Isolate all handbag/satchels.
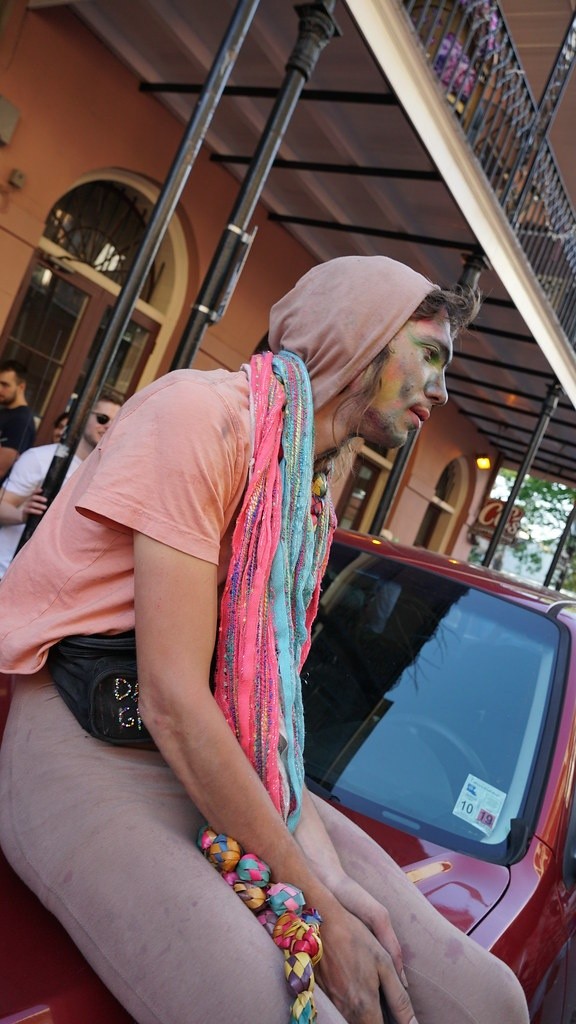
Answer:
[43,630,221,749]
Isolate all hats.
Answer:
[267,254,442,415]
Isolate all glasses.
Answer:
[91,410,113,424]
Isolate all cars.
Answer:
[0,528,576,1023]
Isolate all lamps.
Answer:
[475,453,491,469]
[35,267,54,289]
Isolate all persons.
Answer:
[0,356,127,581]
[0,255,533,1024]
